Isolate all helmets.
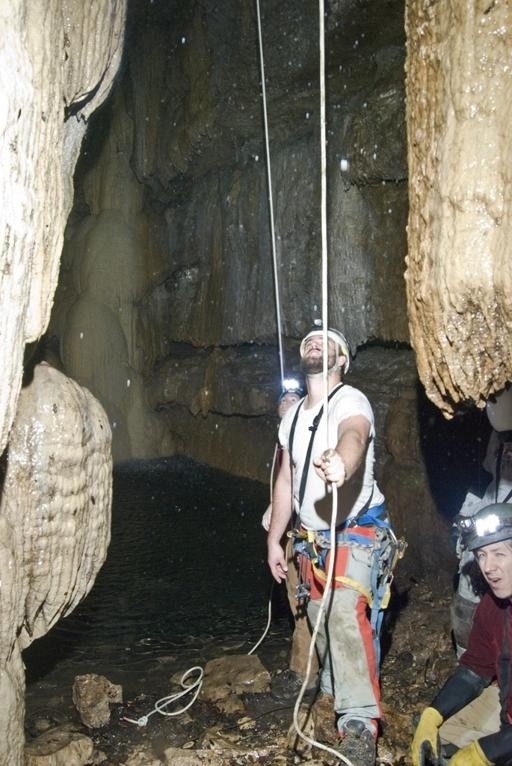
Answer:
[280,387,305,399]
[299,327,351,375]
[451,503,512,551]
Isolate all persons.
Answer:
[266,327,409,766]
[411,502,512,766]
[451,426,511,665]
[261,378,320,702]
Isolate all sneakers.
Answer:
[339,712,378,765]
[313,693,338,743]
[287,703,315,753]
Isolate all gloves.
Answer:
[448,739,496,766]
[410,707,443,766]
